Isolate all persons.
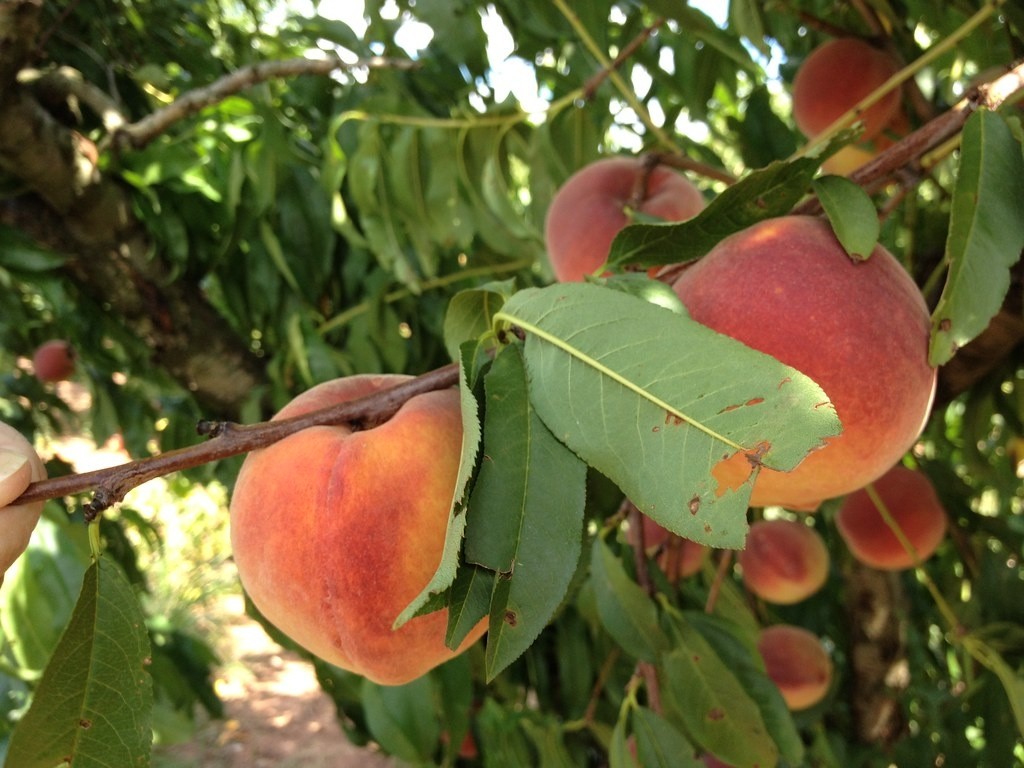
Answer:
[0,420,48,592]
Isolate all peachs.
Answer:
[229,40,948,714]
[34,342,75,383]
